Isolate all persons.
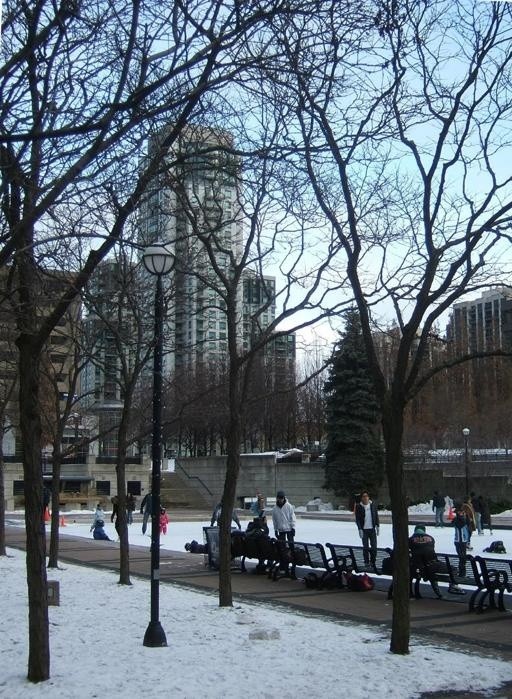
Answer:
[245,511,275,570]
[452,491,494,579]
[409,523,468,601]
[483,541,508,554]
[271,490,298,571]
[210,493,242,531]
[354,491,381,567]
[88,491,169,542]
[432,490,446,528]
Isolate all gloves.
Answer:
[359,529,364,538]
[377,527,379,536]
[276,531,279,538]
[292,528,295,536]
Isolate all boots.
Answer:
[466,542,473,549]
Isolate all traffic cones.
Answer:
[44,506,50,522]
[447,504,455,520]
[59,515,67,528]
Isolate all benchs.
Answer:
[232,532,512,616]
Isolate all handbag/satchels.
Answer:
[305,570,374,591]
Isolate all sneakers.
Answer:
[448,583,467,594]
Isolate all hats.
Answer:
[278,491,285,499]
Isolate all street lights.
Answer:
[462,427,473,498]
[138,239,177,649]
